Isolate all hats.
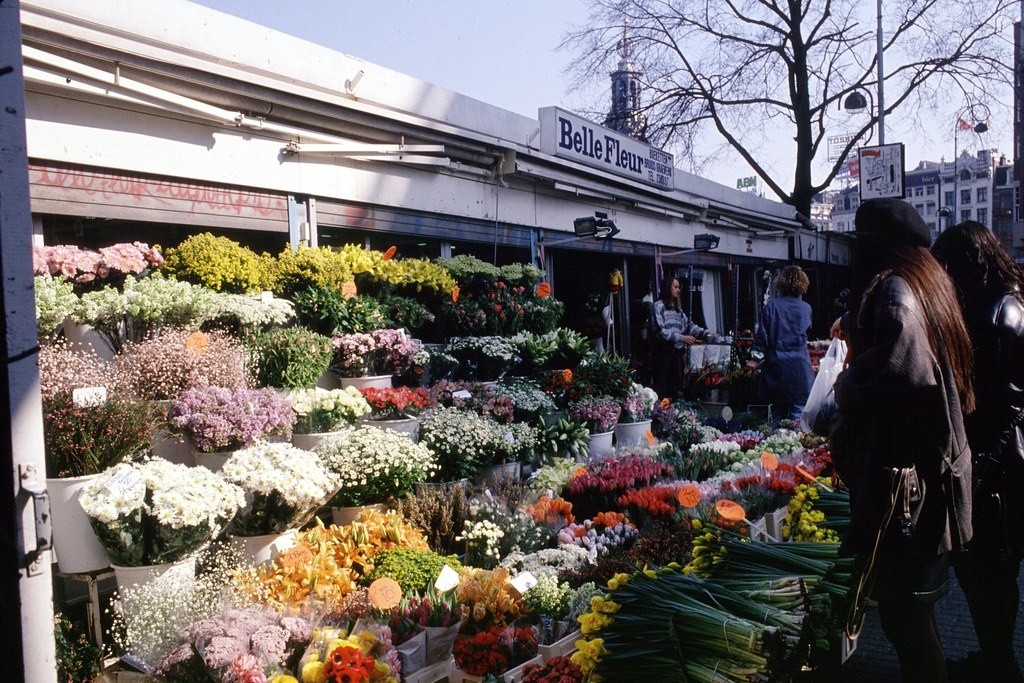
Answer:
[854,197,932,247]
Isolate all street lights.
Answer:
[935,103,990,226]
[838,84,885,146]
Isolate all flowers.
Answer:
[33,230,860,683]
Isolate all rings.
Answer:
[693,338,695,339]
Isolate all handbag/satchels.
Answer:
[799,335,848,438]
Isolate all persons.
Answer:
[754,265,815,423]
[652,276,733,405]
[803,197,1024,683]
[609,266,623,294]
[742,330,762,371]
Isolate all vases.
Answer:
[63,313,126,365]
[413,478,468,496]
[110,556,196,652]
[369,418,420,446]
[537,630,580,661]
[616,419,652,445]
[292,427,352,452]
[743,517,767,543]
[484,462,518,486]
[226,528,300,565]
[48,473,110,576]
[418,344,444,385]
[579,430,614,460]
[191,451,231,471]
[126,317,161,340]
[765,505,793,543]
[147,401,195,467]
[446,656,542,683]
[332,501,384,522]
[339,373,393,392]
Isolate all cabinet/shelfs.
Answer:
[53,560,123,670]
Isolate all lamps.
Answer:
[537,213,620,249]
[656,234,719,270]
